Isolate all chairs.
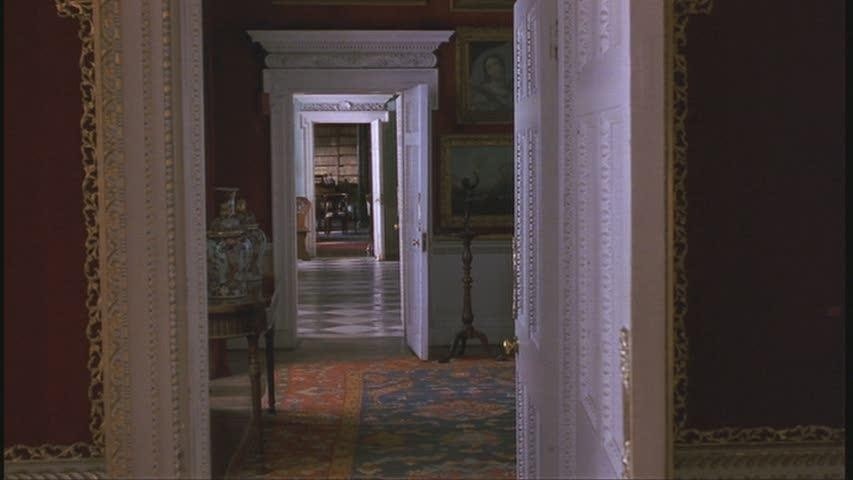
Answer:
[321,193,350,237]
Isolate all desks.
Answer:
[204,275,279,474]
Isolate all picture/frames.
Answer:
[453,26,514,125]
[429,131,515,243]
